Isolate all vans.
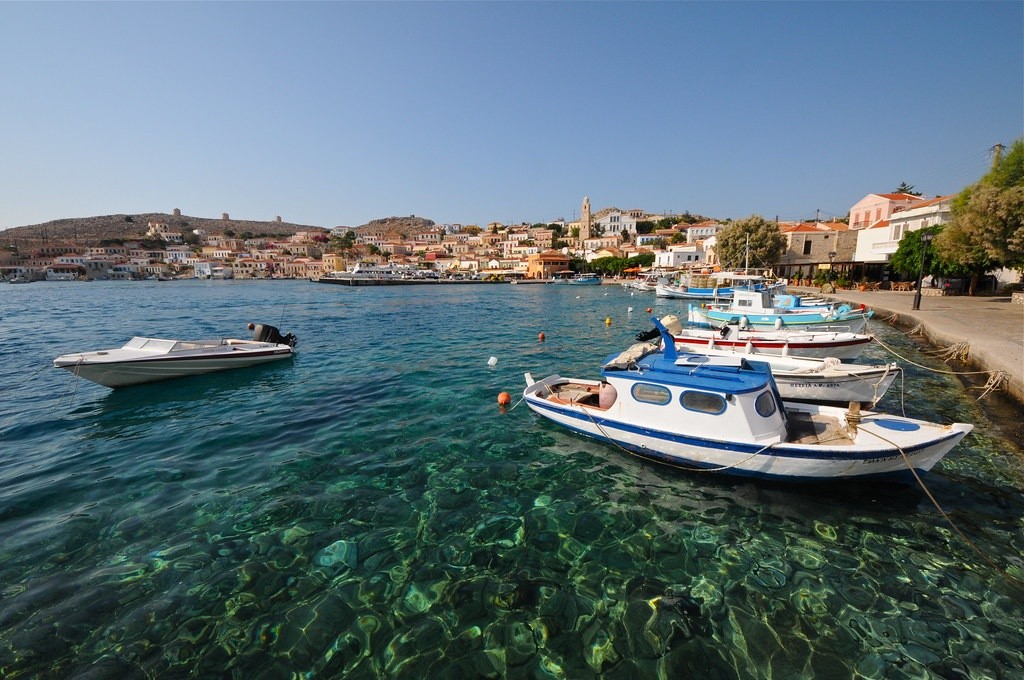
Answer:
[424,273,441,279]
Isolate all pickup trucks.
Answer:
[472,273,489,280]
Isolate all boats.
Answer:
[523,233,974,490]
[53,304,299,388]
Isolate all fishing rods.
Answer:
[274,303,287,346]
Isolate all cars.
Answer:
[450,273,464,280]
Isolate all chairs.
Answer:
[890,281,916,291]
[854,281,882,292]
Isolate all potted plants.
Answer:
[792,269,852,290]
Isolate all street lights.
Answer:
[828,252,836,283]
[912,231,934,311]
